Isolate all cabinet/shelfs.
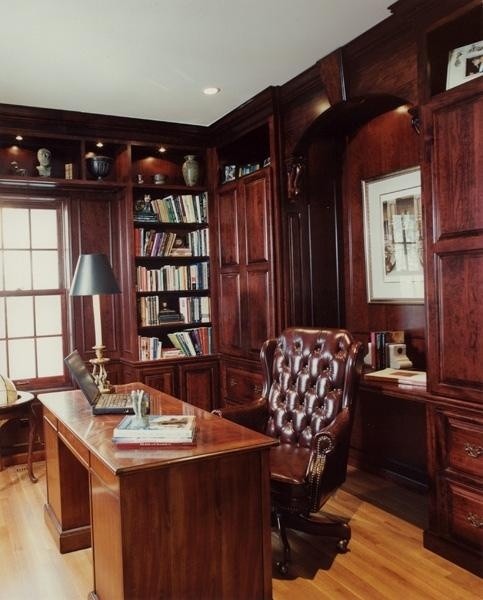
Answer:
[387,0,482,581]
[208,105,337,410]
[115,181,223,416]
[0,127,217,191]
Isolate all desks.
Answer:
[32,381,281,600]
[358,363,429,409]
[0,390,44,486]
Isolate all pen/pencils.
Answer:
[131,389,145,418]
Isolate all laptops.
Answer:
[64,349,150,415]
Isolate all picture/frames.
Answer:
[358,163,425,308]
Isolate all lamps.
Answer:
[68,249,123,395]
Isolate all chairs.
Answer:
[208,323,367,577]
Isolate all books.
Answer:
[111,415,199,448]
[363,331,425,387]
[133,192,213,361]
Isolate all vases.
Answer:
[180,154,202,188]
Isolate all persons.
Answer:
[468,57,481,76]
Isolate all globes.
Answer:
[0,372,38,484]
